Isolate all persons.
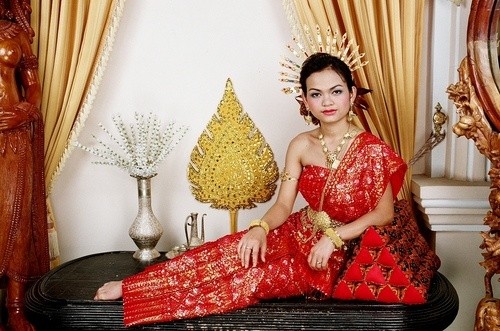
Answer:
[93,22,407,328]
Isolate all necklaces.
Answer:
[318,124,360,167]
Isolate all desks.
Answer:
[24,250,458,331]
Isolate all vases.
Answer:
[130,173,162,261]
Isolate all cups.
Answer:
[172,244,188,257]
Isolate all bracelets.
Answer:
[248,219,270,236]
[325,227,345,249]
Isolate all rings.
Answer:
[245,246,253,250]
[317,261,322,264]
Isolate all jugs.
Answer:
[183,212,207,251]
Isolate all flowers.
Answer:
[74,112,189,174]
